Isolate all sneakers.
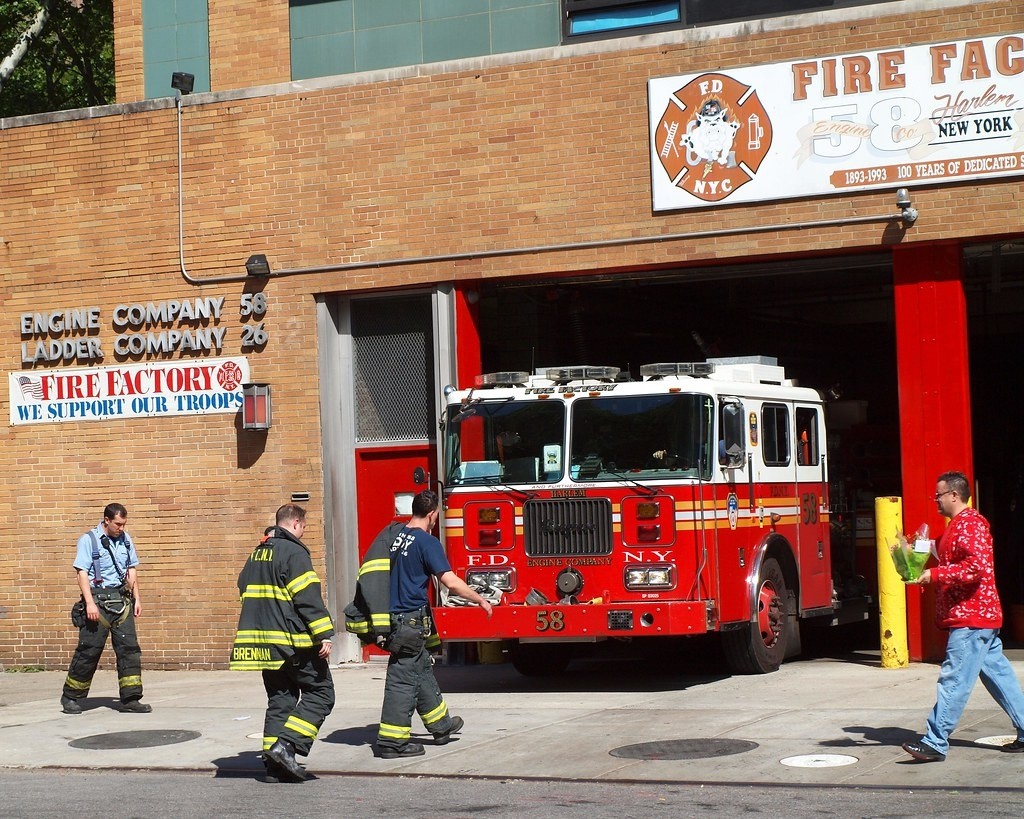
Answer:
[1001,740,1024,753]
[902,740,946,761]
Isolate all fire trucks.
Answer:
[412,356,901,679]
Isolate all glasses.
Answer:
[935,490,954,498]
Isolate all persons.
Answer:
[903,471,1024,761]
[230,503,336,783]
[652,420,728,470]
[345,491,492,759]
[60,502,154,714]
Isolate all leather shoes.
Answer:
[119,699,153,712]
[61,694,82,714]
[264,737,307,781]
[432,716,464,745]
[264,766,316,783]
[381,743,425,758]
